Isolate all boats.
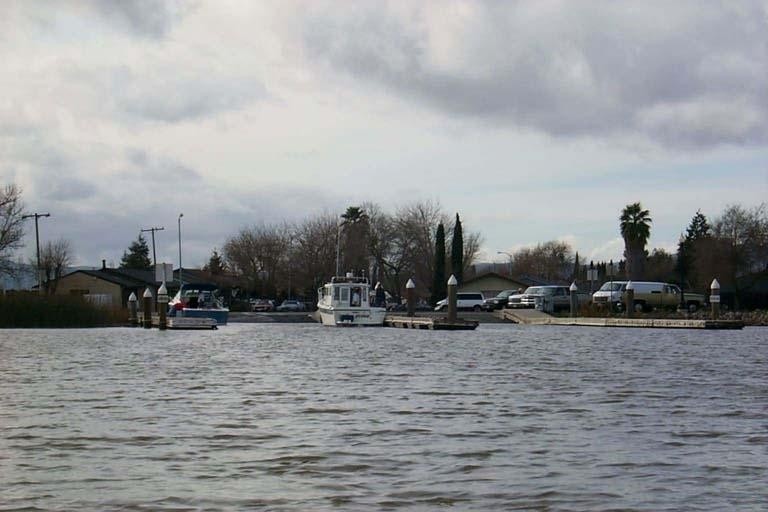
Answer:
[168,284,229,325]
[308,270,386,326]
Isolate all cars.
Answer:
[405,297,431,311]
[434,285,592,312]
[249,298,307,312]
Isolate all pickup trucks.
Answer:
[621,284,707,313]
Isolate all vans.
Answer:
[592,281,677,313]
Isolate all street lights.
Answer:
[288,235,293,300]
[498,252,512,276]
[22,213,50,290]
[140,227,164,267]
[608,259,614,312]
[178,214,184,285]
